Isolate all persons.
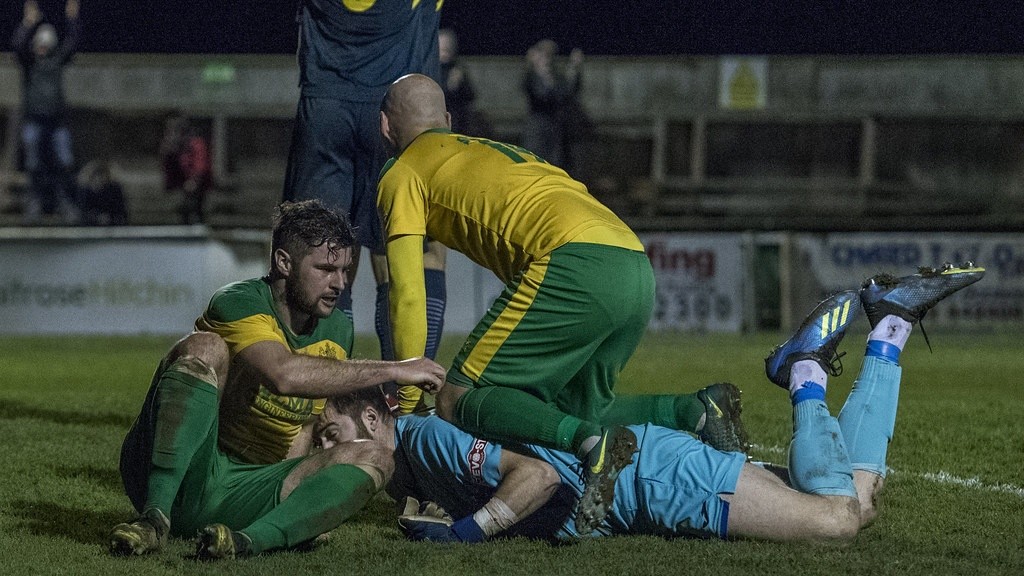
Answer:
[106,199,447,560]
[374,73,755,536]
[13,0,89,227]
[161,113,211,227]
[308,259,986,544]
[283,0,446,422]
[430,30,481,131]
[78,160,134,225]
[519,40,597,189]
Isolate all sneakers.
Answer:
[857,262,985,354]
[106,508,170,556]
[196,523,251,562]
[567,425,640,535]
[697,382,754,461]
[764,290,861,390]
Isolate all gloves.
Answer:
[397,495,489,542]
